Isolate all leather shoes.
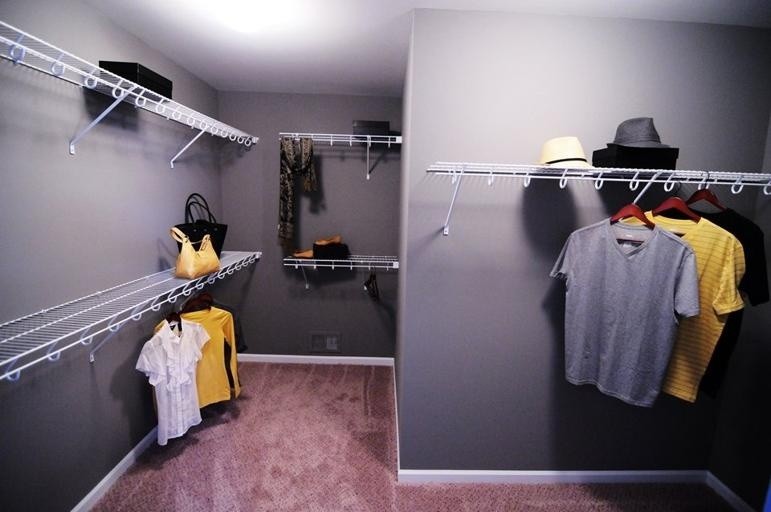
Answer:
[314,234,342,246]
[291,250,315,259]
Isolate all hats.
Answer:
[606,117,671,150]
[531,136,597,171]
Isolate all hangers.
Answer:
[574,170,763,248]
[144,283,238,348]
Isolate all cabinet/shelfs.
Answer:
[1,20,264,381]
[279,132,403,272]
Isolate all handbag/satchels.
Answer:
[170,226,221,279]
[170,193,229,260]
